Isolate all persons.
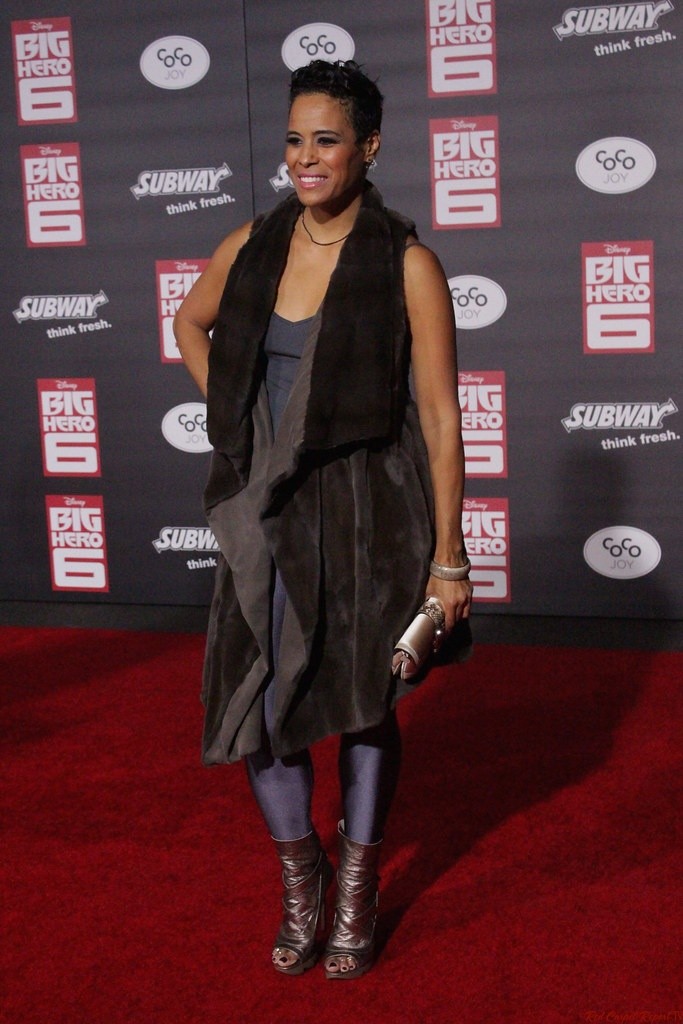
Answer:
[172,61,477,976]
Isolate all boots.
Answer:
[321,819,382,979]
[271,828,333,976]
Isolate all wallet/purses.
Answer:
[392,597,445,679]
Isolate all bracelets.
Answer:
[429,557,472,580]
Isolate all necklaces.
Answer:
[301,206,355,246]
